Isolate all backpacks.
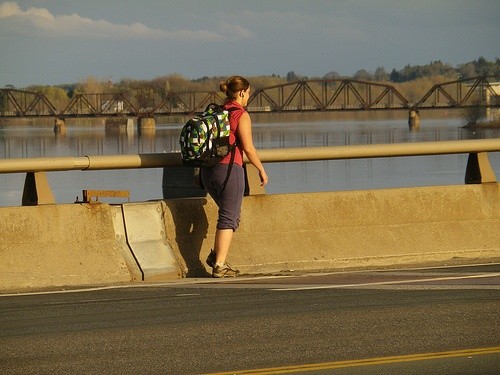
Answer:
[180,103,242,193]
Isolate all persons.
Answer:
[200,75,269,279]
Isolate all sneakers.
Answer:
[212,263,240,278]
[206,248,231,269]
